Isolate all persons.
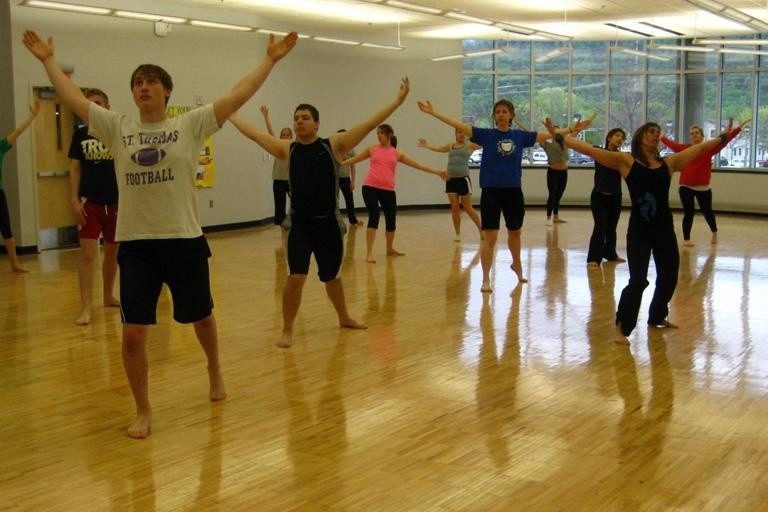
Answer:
[416,100,581,293]
[537,228,571,318]
[585,261,618,396]
[658,118,753,247]
[260,104,293,230]
[476,283,528,469]
[68,89,125,326]
[543,116,734,346]
[512,114,597,226]
[226,78,410,349]
[339,124,449,263]
[19,30,299,439]
[611,325,689,511]
[667,247,716,378]
[567,128,632,267]
[0,95,43,274]
[335,129,364,226]
[445,244,481,350]
[417,128,484,241]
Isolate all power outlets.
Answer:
[208,199,214,208]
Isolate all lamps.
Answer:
[596,0,767,66]
[421,0,573,65]
[15,0,405,54]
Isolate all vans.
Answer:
[531,151,548,161]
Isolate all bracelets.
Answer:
[568,127,573,133]
[719,133,727,143]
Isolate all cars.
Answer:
[568,150,593,164]
[468,148,483,163]
[711,155,727,165]
[758,159,767,166]
